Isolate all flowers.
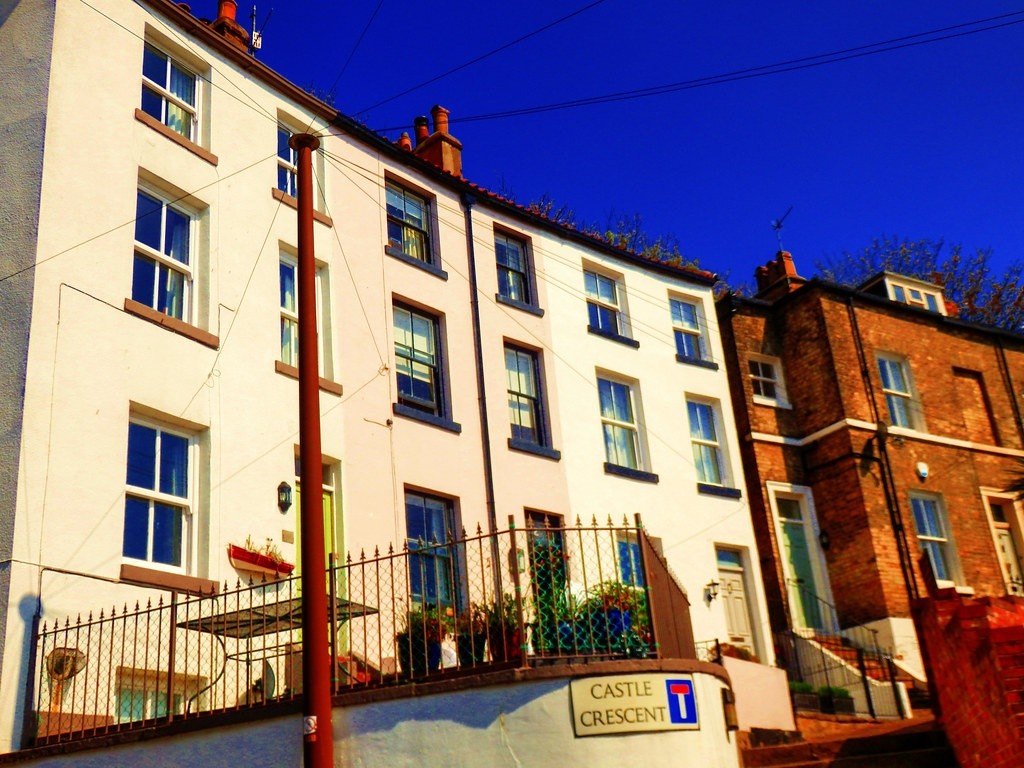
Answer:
[396,582,531,642]
[578,582,652,645]
[528,539,569,617]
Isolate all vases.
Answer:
[456,635,489,666]
[529,618,578,657]
[583,607,638,646]
[488,623,529,662]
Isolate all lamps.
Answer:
[509,548,526,574]
[819,529,830,550]
[278,482,292,512]
[705,580,719,601]
[868,420,889,443]
[251,679,262,703]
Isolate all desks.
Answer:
[175,594,379,715]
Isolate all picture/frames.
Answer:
[398,641,442,673]
